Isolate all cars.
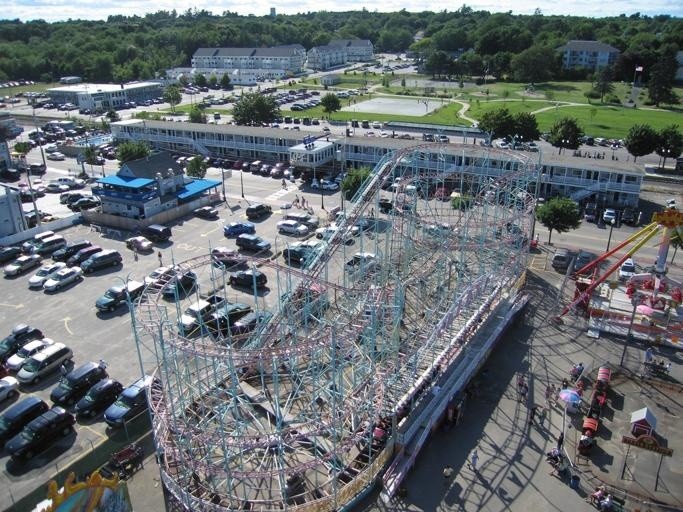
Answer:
[552,248,593,273]
[450,186,637,227]
[619,258,635,281]
[531,240,537,253]
[480,135,625,152]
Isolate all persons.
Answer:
[643,346,673,376]
[99,358,110,373]
[517,362,606,427]
[157,250,164,267]
[58,362,67,383]
[443,464,454,484]
[292,194,307,210]
[545,432,570,481]
[536,233,539,240]
[132,241,139,257]
[376,413,392,435]
[282,177,287,191]
[466,448,480,473]
[588,485,612,512]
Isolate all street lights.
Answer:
[606,219,615,251]
